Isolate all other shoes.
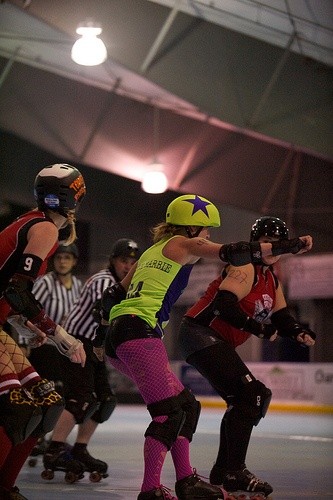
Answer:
[0,486,30,500]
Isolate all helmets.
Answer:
[250,216,288,240]
[33,163,85,212]
[54,241,78,258]
[166,195,220,229]
[109,239,141,259]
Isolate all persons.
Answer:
[0,163,88,500]
[178,216,317,493]
[22,243,83,387]
[42,239,139,482]
[105,195,312,500]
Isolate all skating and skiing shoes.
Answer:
[209,462,226,487]
[222,464,273,500]
[28,437,50,467]
[69,448,108,482]
[40,446,83,483]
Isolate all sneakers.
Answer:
[137,484,177,500]
[174,468,225,500]
